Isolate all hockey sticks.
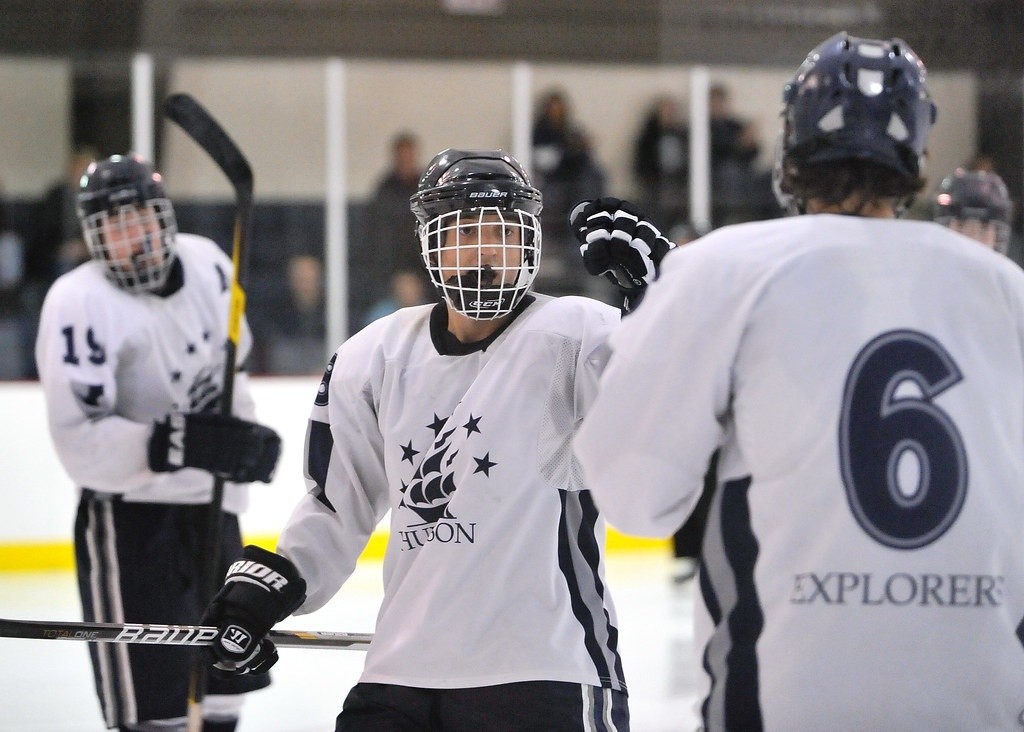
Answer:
[162,91,255,732]
[0,618,375,650]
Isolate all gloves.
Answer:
[197,545,308,676]
[147,413,281,484]
[571,197,681,321]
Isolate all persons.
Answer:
[710,85,758,208]
[638,101,689,205]
[570,30,1024,732]
[934,169,1014,252]
[35,153,281,732]
[531,88,590,220]
[31,148,92,278]
[199,147,678,732]
[357,132,437,326]
[253,255,324,377]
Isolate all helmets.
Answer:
[771,31,937,219]
[74,152,178,295]
[934,166,1014,255]
[409,147,544,322]
